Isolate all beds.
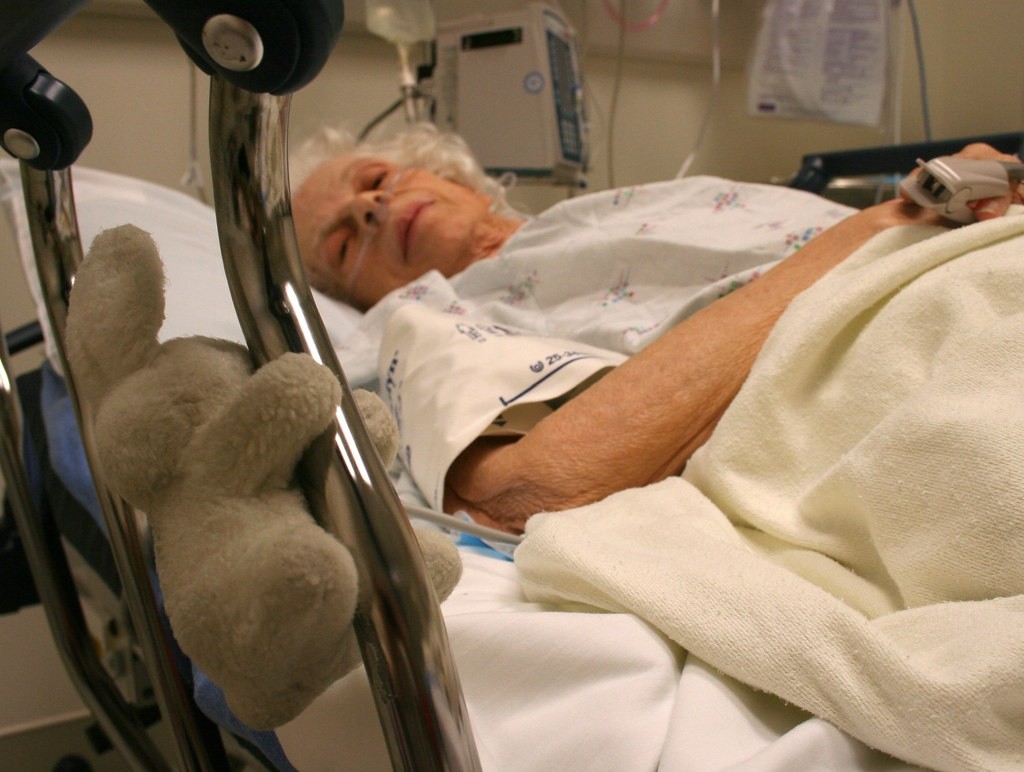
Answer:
[0,0,1024,772]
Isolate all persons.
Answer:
[282,126,1024,540]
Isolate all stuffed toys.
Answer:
[64,224,463,730]
